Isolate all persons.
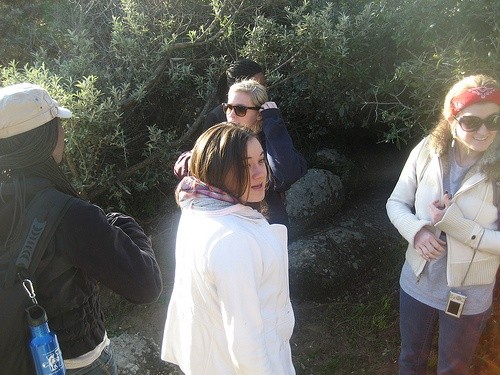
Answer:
[0,84,162,375]
[176,61,266,157]
[384,73,500,375]
[159,122,298,375]
[220,79,307,239]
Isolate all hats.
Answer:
[0,83,72,138]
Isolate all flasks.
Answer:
[25,305,66,375]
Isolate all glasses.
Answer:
[222,103,261,117]
[454,113,500,131]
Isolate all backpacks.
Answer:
[0,187,74,375]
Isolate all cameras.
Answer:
[445,291,466,318]
